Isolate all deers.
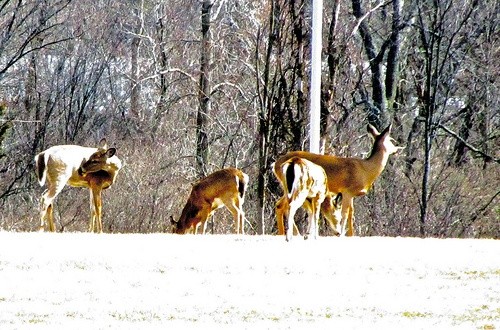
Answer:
[169,167,250,234]
[273,124,398,242]
[34,137,122,234]
